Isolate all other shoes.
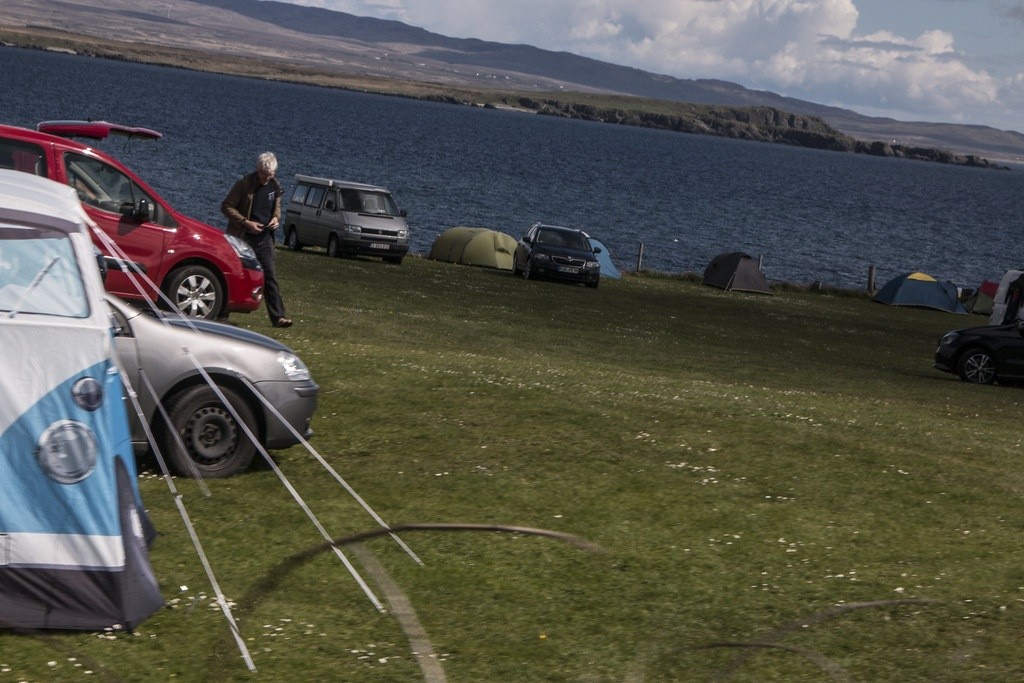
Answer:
[273,317,293,328]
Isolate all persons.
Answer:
[218,151,293,328]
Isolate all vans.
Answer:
[282,174,411,265]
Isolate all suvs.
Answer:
[511,222,602,288]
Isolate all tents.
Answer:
[428,226,517,271]
[945,269,1024,326]
[1,165,171,628]
[871,272,969,315]
[700,251,773,295]
[578,237,624,281]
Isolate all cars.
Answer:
[931,319,1024,384]
[0,123,267,323]
[103,288,324,482]
